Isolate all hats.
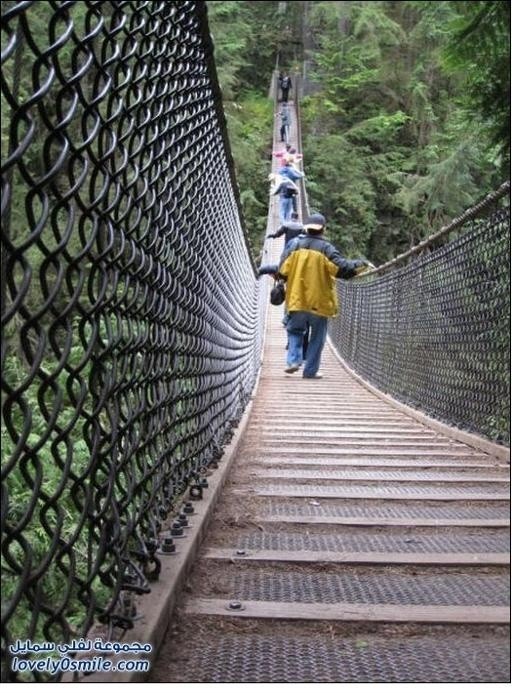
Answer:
[304,213,325,230]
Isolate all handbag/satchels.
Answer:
[271,276,285,305]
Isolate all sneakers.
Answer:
[284,364,297,373]
[303,375,322,379]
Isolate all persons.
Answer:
[263,211,307,247]
[275,210,375,379]
[255,263,310,360]
[268,65,306,223]
